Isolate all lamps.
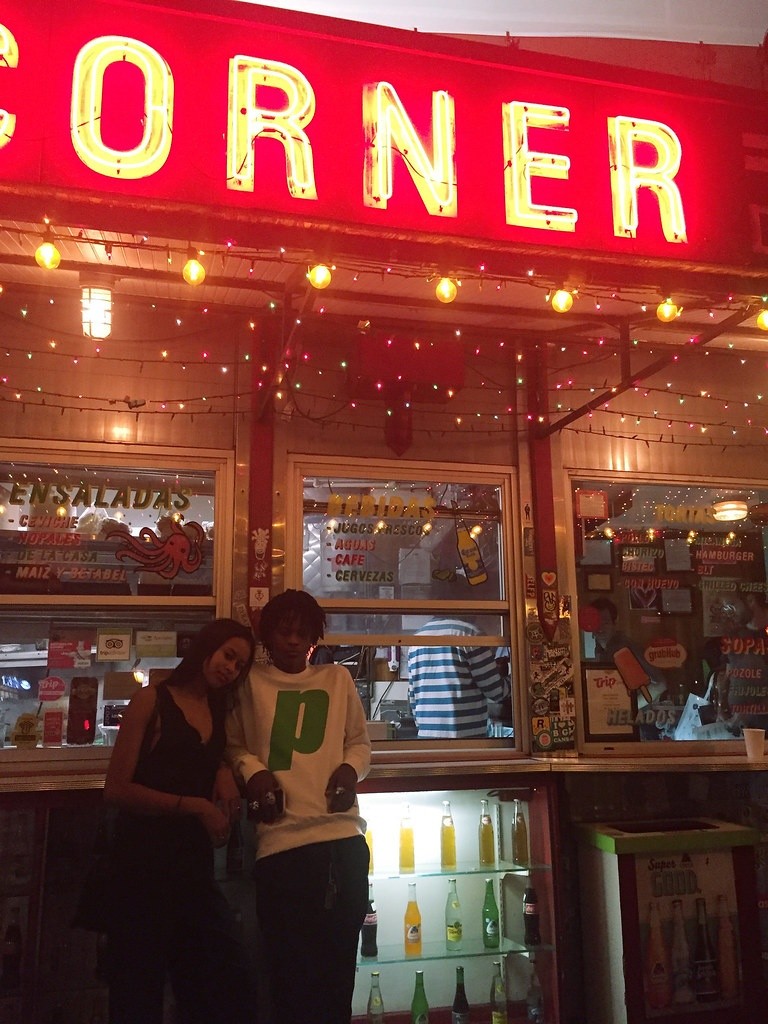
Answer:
[76,270,117,341]
[713,499,748,521]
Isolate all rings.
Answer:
[335,787,346,795]
[249,801,259,811]
[236,806,240,810]
[264,792,276,805]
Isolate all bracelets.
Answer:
[175,794,183,817]
[216,831,226,839]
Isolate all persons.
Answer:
[102,617,256,1024]
[408,582,511,739]
[590,595,644,663]
[716,577,768,740]
[226,587,372,1024]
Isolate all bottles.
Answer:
[451,966,472,1024]
[361,883,378,957]
[440,800,457,871]
[411,970,429,1024]
[478,799,495,866]
[490,961,508,1024]
[525,962,545,1024]
[695,894,740,1002]
[398,803,415,874]
[482,878,500,948]
[649,860,698,898]
[452,507,488,585]
[364,818,375,876]
[523,875,542,946]
[404,883,423,956]
[445,879,464,951]
[646,901,672,1009]
[511,798,529,866]
[670,899,697,1005]
[367,971,385,1024]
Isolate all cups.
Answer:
[743,729,765,761]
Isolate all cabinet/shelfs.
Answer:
[349,770,568,1024]
